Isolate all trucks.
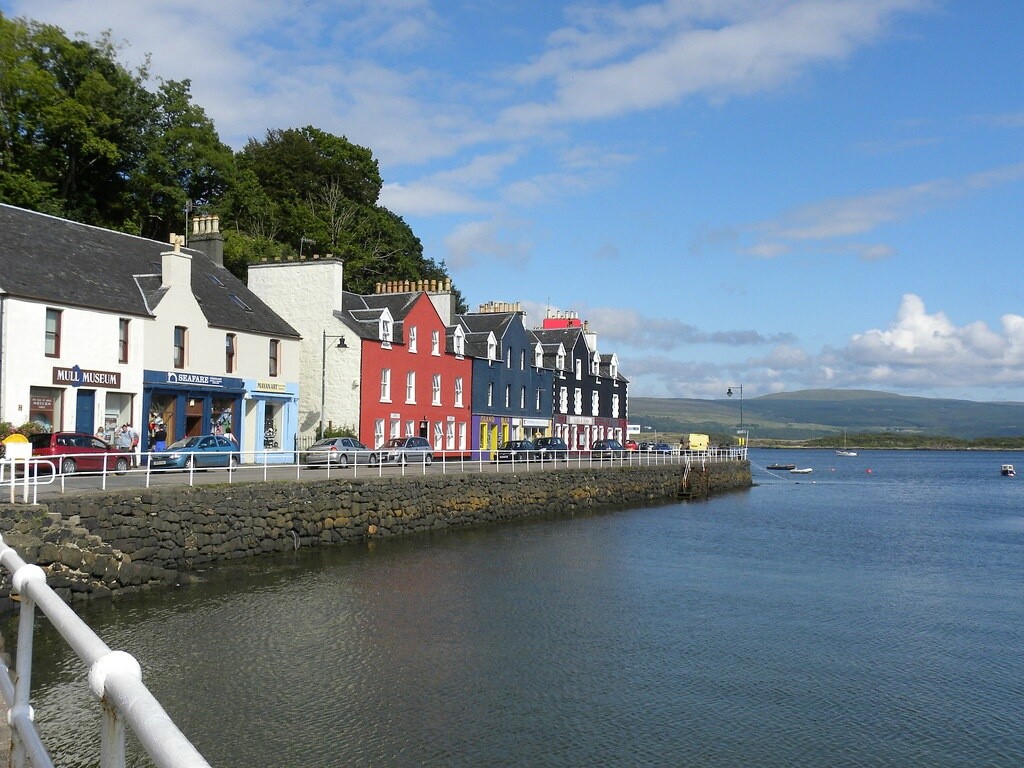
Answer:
[679,433,710,455]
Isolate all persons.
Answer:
[224,428,239,446]
[215,429,224,443]
[155,424,167,452]
[94,427,106,446]
[115,422,138,469]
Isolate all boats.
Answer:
[790,468,813,474]
[1000,464,1017,477]
[766,463,796,470]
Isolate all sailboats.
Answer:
[835,425,859,456]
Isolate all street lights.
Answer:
[726,385,744,446]
[320,330,348,438]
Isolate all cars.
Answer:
[532,436,568,462]
[375,437,434,466]
[637,441,672,455]
[625,440,639,454]
[305,436,379,470]
[150,434,240,473]
[13,430,132,479]
[590,439,627,462]
[490,440,539,463]
